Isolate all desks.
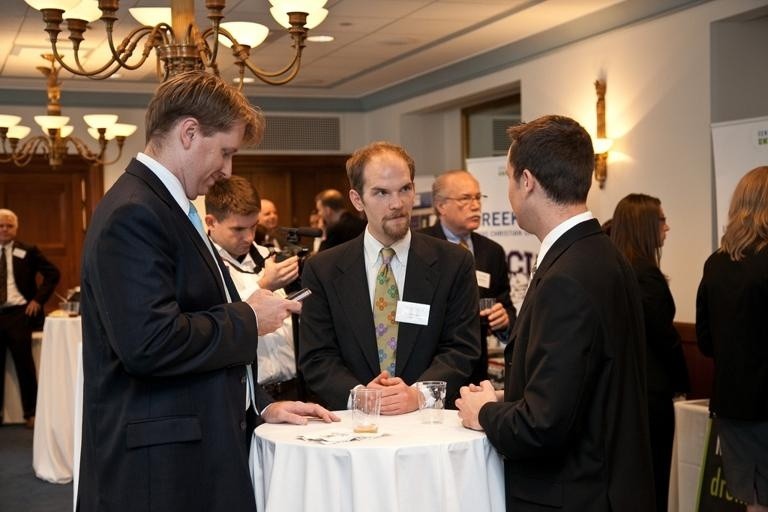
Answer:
[41,308,83,482]
[665,398,712,512]
[250,407,494,512]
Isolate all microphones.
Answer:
[280,227,323,237]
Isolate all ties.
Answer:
[458,238,467,248]
[1,250,8,307]
[188,202,261,419]
[375,249,400,375]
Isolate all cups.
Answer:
[350,389,380,432]
[479,298,496,326]
[416,381,447,423]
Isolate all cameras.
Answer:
[274,243,310,279]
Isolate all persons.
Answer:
[296,140,481,416]
[72,69,305,512]
[204,177,368,398]
[414,170,518,344]
[0,208,62,430]
[693,165,768,512]
[455,115,690,511]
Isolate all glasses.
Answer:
[447,194,487,204]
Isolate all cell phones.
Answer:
[285,287,312,301]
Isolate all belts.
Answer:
[258,375,298,395]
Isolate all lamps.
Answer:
[0,54,139,169]
[586,78,610,191]
[23,0,329,97]
